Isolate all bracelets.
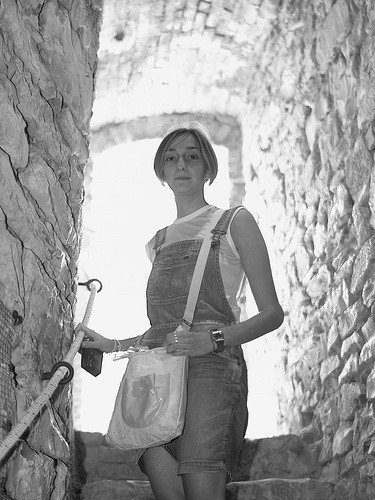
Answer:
[110,339,122,352]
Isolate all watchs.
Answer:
[208,327,226,352]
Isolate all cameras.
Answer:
[80,337,103,377]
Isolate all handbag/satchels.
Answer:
[106,343,187,450]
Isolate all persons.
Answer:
[74,122,284,500]
